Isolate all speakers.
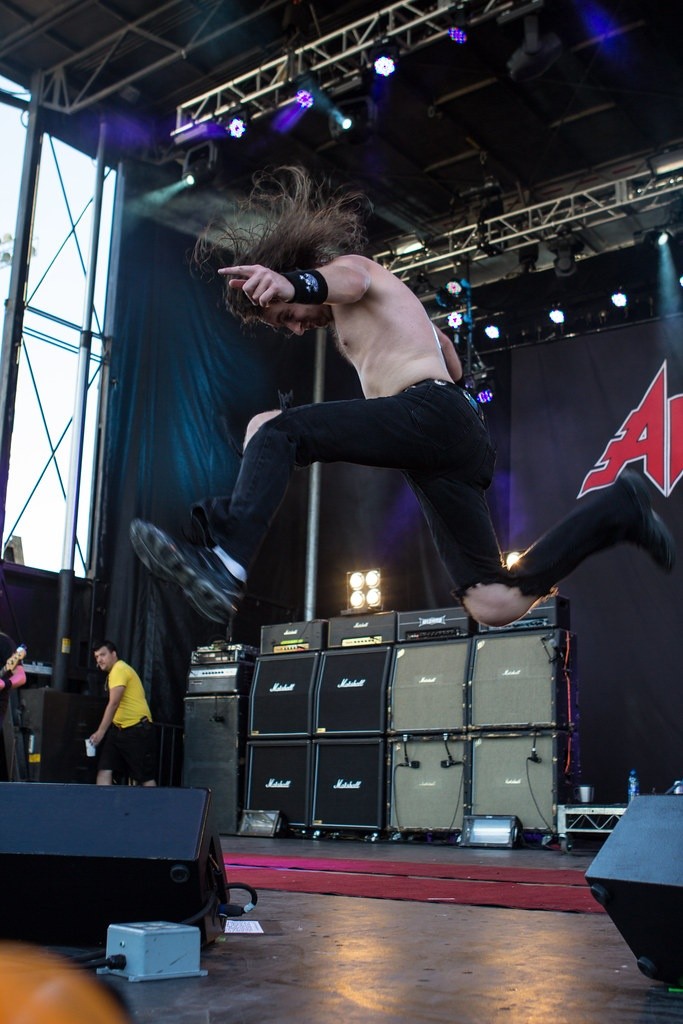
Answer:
[0,779,231,948]
[181,694,249,836]
[585,795,683,988]
[250,627,579,836]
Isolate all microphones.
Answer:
[528,755,542,764]
[441,759,462,767]
[210,715,224,722]
[398,760,420,769]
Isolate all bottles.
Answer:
[627,769,639,805]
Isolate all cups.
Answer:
[573,785,593,803]
[84,739,96,757]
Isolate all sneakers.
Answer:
[130,520,246,623]
[618,470,676,569]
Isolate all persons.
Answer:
[0,632,27,782]
[128,166,674,627]
[88,640,158,787]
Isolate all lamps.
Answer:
[227,102,252,142]
[457,814,522,848]
[548,236,585,277]
[180,139,225,189]
[339,567,384,616]
[327,96,384,150]
[448,12,472,44]
[372,37,404,78]
[501,548,526,571]
[436,277,471,309]
[295,70,325,114]
[507,12,564,84]
[236,809,284,840]
[471,366,498,410]
[550,307,565,338]
[484,323,511,348]
[611,287,630,322]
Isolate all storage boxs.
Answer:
[557,802,629,854]
